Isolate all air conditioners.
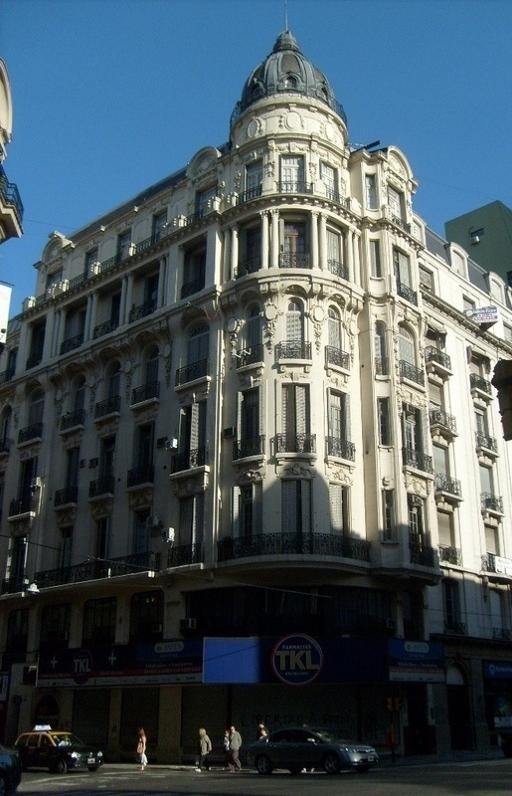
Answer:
[165,436,178,451]
[152,623,164,634]
[159,526,176,542]
[179,617,196,632]
[223,425,236,439]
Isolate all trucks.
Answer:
[493,715,512,759]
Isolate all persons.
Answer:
[229,725,243,770]
[137,727,148,770]
[194,727,212,773]
[300,723,315,773]
[257,720,270,743]
[223,729,231,770]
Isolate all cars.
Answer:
[245,726,382,776]
[13,725,102,773]
[0,743,23,793]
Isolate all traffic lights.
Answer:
[396,696,401,708]
[383,696,393,711]
